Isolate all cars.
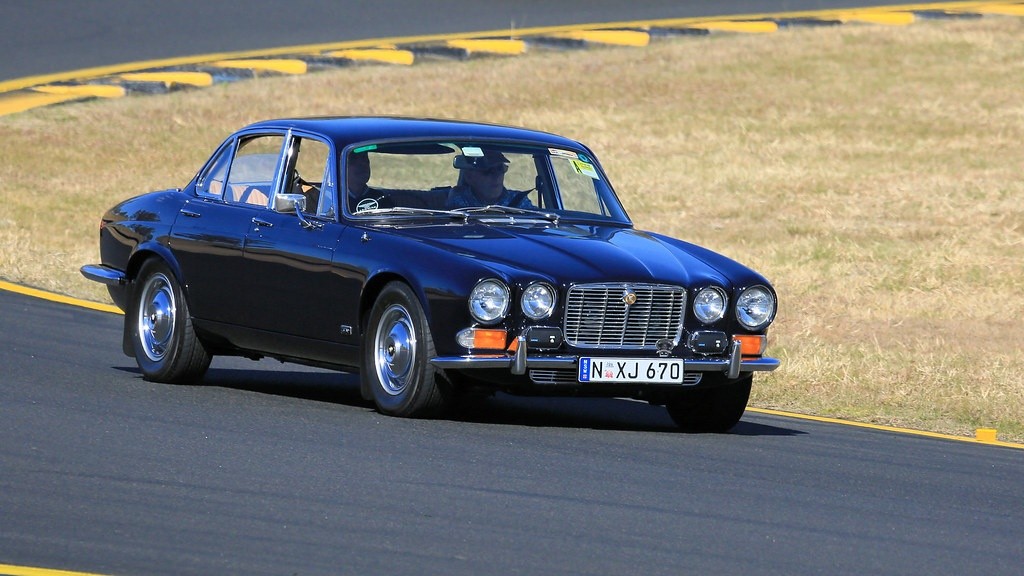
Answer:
[78,115,782,433]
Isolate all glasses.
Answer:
[484,165,508,176]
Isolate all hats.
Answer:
[478,151,510,165]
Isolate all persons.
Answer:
[447,145,534,207]
[305,148,390,217]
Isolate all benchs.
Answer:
[207,180,450,214]
